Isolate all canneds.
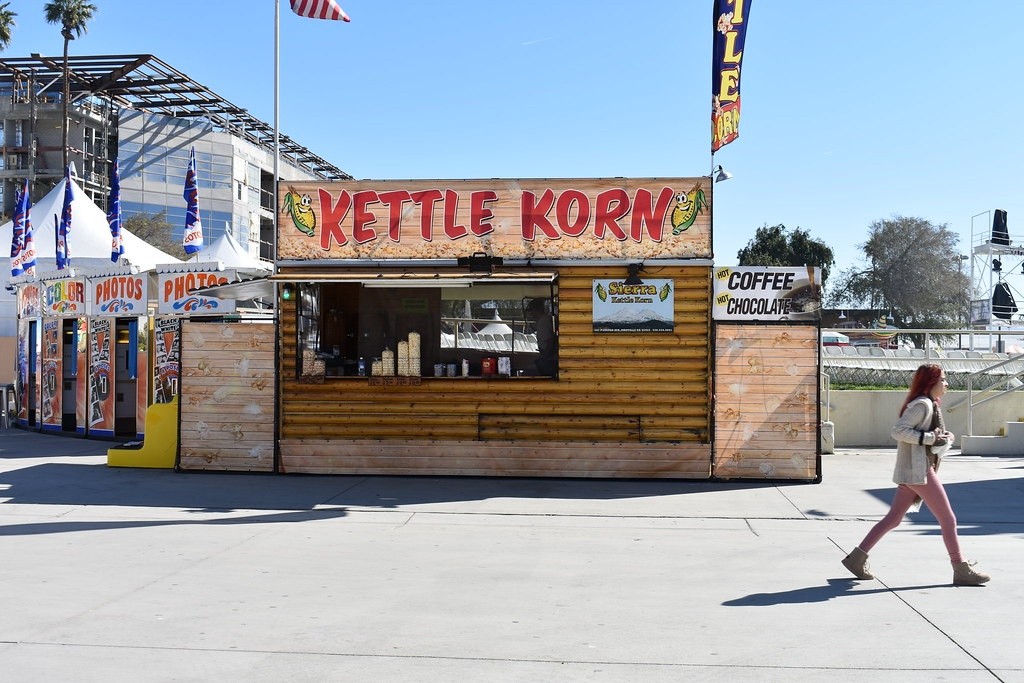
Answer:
[434,364,444,377]
[447,364,456,377]
[498,356,511,376]
[481,357,496,376]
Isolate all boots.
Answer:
[952,560,991,586]
[841,547,873,579]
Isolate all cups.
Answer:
[498,356,511,376]
[434,365,443,377]
[480,358,496,376]
[447,364,456,377]
[462,359,469,377]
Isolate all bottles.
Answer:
[358,357,365,376]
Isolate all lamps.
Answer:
[469,250,492,275]
[622,264,644,285]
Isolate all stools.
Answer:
[0,383,17,429]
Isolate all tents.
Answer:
[821,327,849,347]
[0,178,275,338]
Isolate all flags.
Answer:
[183,147,204,255]
[55,168,74,270]
[290,0,350,23]
[12,189,25,277]
[20,177,37,278]
[713,0,752,154]
[107,158,124,262]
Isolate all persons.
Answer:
[841,364,990,585]
[529,300,557,376]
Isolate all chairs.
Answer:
[822,345,1024,391]
